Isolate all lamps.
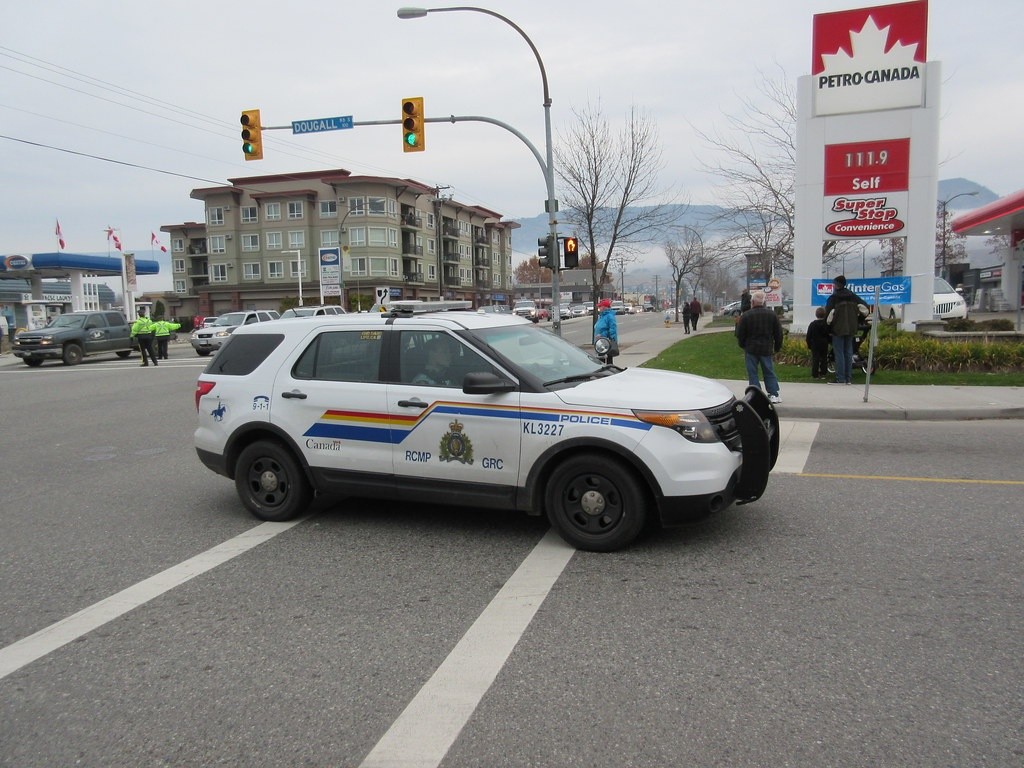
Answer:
[65,272,71,280]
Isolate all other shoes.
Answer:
[813,375,819,381]
[155,363,158,366]
[827,378,851,385]
[142,363,148,366]
[821,375,826,380]
[687,331,690,334]
[685,330,687,334]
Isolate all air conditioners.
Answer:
[223,205,231,210]
[226,262,234,268]
[339,227,346,232]
[225,234,232,240]
[338,197,345,203]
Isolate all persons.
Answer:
[741,289,752,314]
[690,297,701,331]
[593,299,618,365]
[149,315,181,359]
[735,291,784,402]
[129,310,158,366]
[47,316,52,325]
[826,275,868,386]
[956,284,967,300]
[682,302,692,334]
[805,307,831,381]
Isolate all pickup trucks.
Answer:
[12,308,179,367]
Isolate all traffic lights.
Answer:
[537,235,553,269]
[239,109,264,161]
[401,96,426,153]
[563,236,579,268]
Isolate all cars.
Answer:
[718,299,794,319]
[470,293,654,324]
[200,316,218,329]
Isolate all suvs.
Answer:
[276,303,348,320]
[191,299,781,555]
[189,309,281,356]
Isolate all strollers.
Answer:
[823,316,878,376]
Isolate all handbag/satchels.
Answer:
[598,338,619,356]
[858,303,869,319]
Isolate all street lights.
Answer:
[942,190,979,280]
[337,197,387,312]
[396,5,562,338]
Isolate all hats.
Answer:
[596,300,610,308]
[138,310,146,314]
[833,275,846,285]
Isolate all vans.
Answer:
[933,276,969,322]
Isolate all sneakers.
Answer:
[769,392,782,403]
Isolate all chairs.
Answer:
[441,335,468,386]
[405,346,424,384]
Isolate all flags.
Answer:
[108,228,121,250]
[56,221,65,249]
[150,233,167,253]
